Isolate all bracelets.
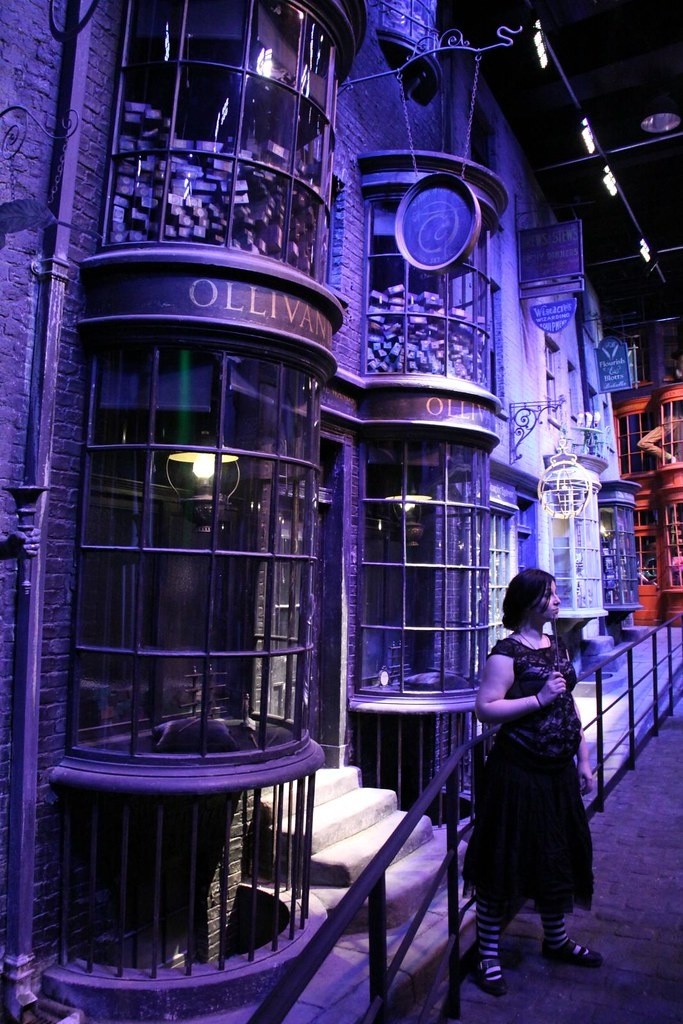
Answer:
[536,694,542,706]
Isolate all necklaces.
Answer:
[519,633,549,649]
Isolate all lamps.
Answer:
[577,116,596,155]
[522,18,549,72]
[385,471,432,546]
[165,397,241,534]
[601,165,618,197]
[640,80,682,134]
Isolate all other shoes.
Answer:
[544,940,602,967]
[472,947,507,997]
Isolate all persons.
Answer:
[461,568,602,994]
